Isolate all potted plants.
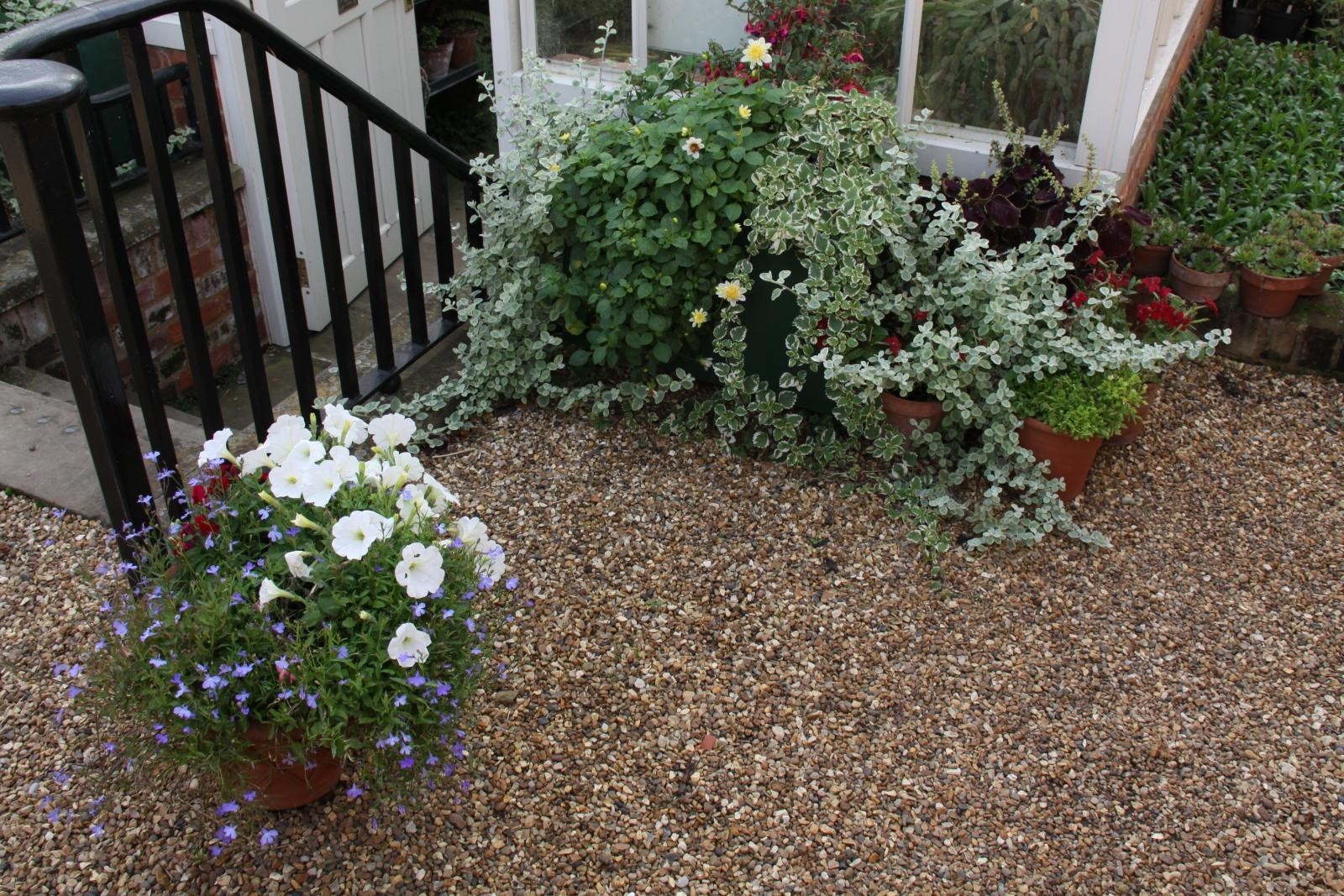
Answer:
[1234,222,1313,320]
[1167,232,1232,307]
[1019,356,1134,502]
[1129,215,1176,277]
[1269,209,1344,296]
[0,0,152,181]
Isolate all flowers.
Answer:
[439,1,1232,569]
[35,398,517,867]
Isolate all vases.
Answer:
[1105,378,1161,446]
[742,246,829,411]
[192,709,341,813]
[881,387,941,450]
